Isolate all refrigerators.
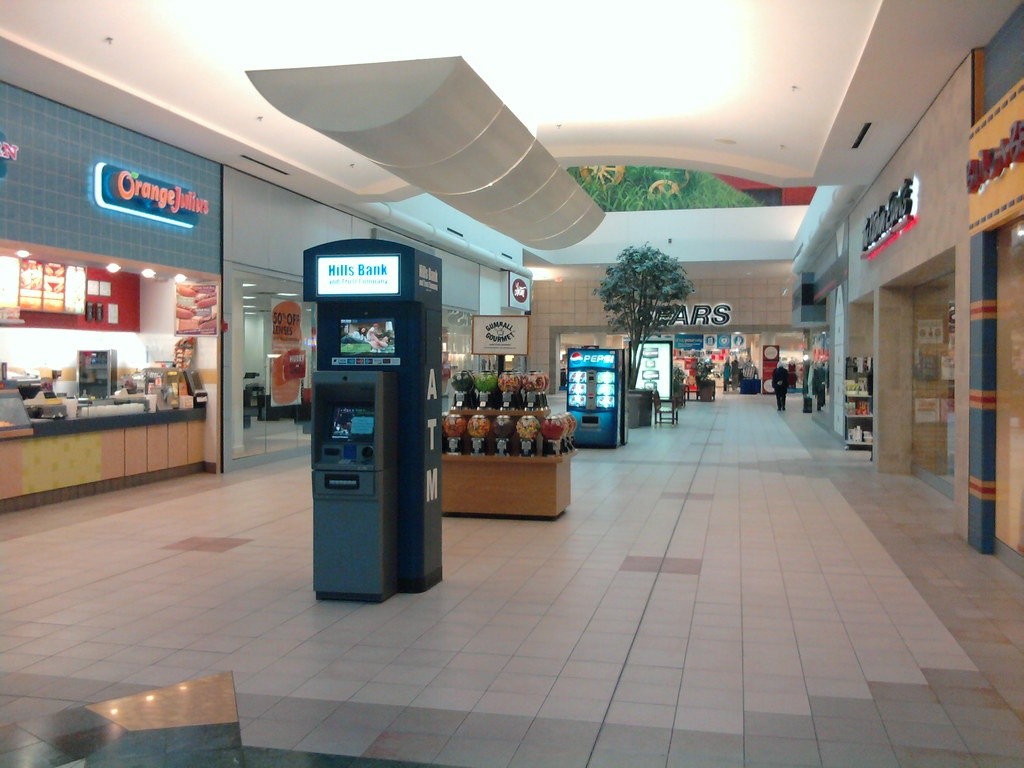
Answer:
[75,349,117,400]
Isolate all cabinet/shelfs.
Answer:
[441,315,578,516]
[844,356,873,450]
[76,350,117,401]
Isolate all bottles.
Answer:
[80,390,87,398]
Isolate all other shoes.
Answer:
[777,408,781,411]
[782,408,785,411]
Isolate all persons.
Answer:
[348,323,390,350]
[788,356,797,389]
[772,362,790,411]
[724,362,730,395]
[813,361,825,412]
[803,354,810,395]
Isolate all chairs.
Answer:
[653,390,678,425]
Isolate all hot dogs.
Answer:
[176,286,218,330]
[43,265,65,292]
